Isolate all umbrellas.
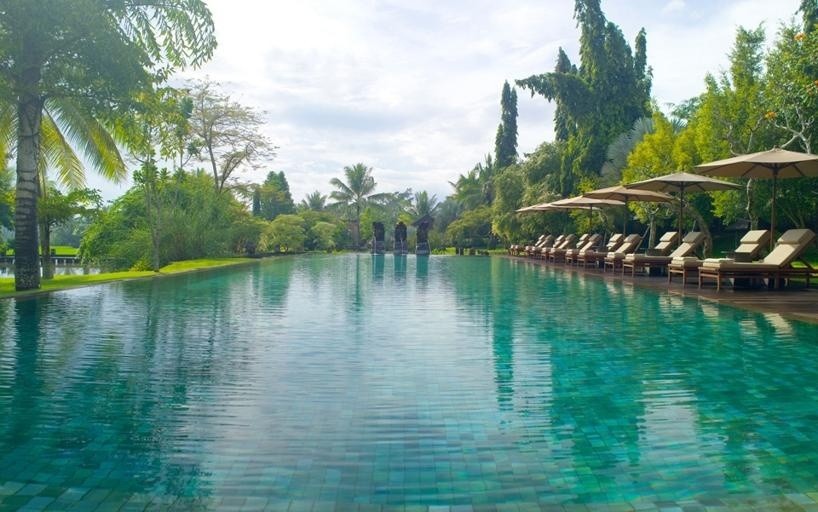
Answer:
[515,194,626,236]
[622,172,744,247]
[693,144,818,252]
[581,185,676,238]
